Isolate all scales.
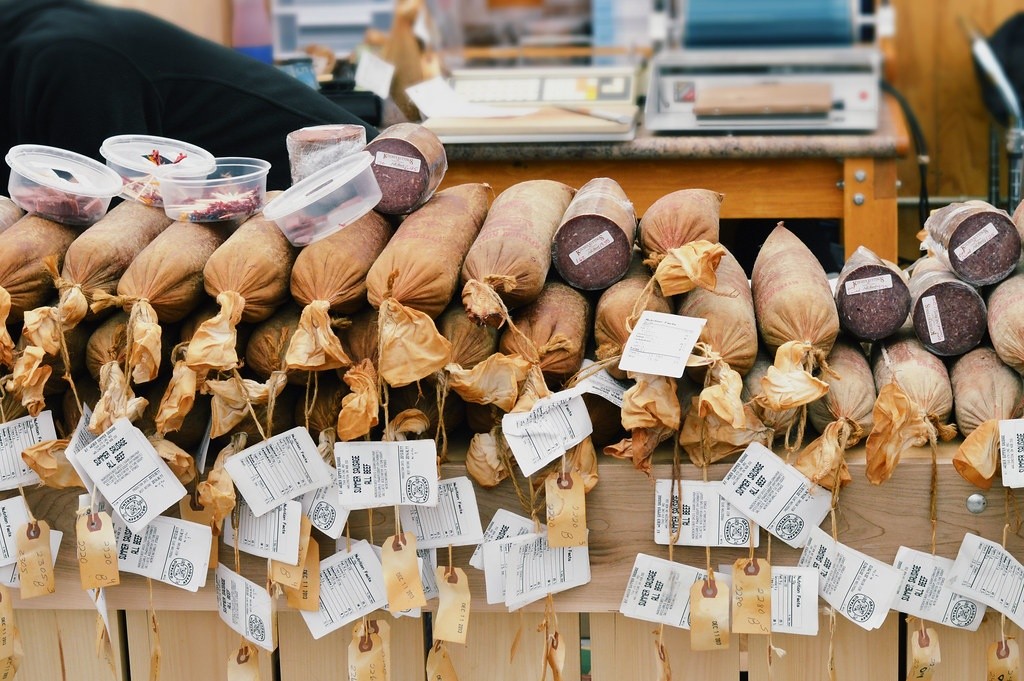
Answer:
[401,44,655,145]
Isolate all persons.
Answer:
[0,0,381,200]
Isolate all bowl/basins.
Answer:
[99,134,216,208]
[263,150,385,248]
[4,143,125,226]
[155,156,272,223]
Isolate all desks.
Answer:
[435,95,910,266]
